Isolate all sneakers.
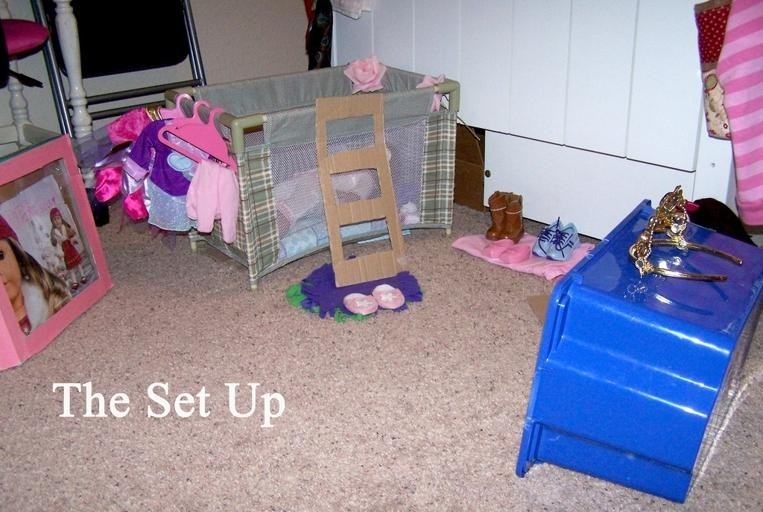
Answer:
[532,217,581,263]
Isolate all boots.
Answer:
[487,191,526,244]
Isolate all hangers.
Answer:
[157,92,238,173]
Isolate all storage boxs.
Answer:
[516,199,763,504]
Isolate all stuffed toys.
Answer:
[270,141,403,239]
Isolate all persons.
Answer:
[0,215,71,336]
[49,207,88,290]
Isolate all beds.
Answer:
[166,61,464,293]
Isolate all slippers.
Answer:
[344,293,378,314]
[372,284,405,310]
